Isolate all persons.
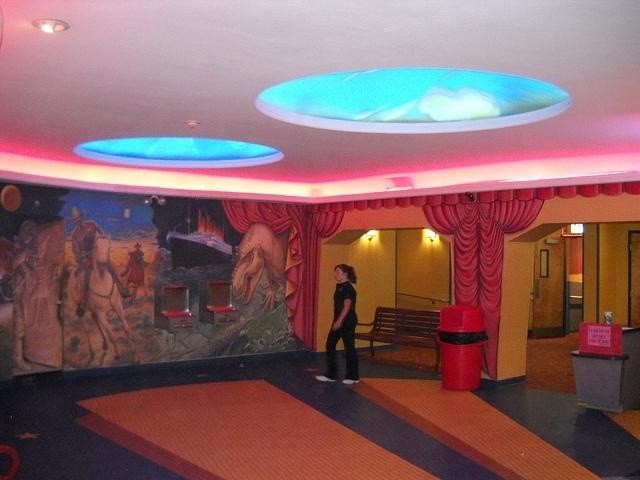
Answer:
[71,206,131,316]
[121,240,150,280]
[315,263,360,385]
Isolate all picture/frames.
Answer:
[539,249,549,278]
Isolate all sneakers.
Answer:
[315,375,336,382]
[341,379,360,384]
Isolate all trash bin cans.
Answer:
[435,304,489,391]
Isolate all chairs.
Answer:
[342,306,441,376]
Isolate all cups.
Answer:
[604,311,615,324]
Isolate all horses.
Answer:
[68,230,141,365]
